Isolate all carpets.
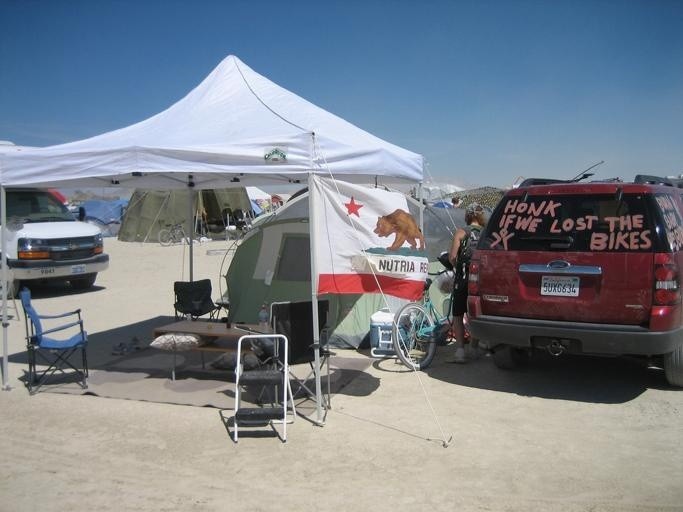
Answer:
[27,315,294,416]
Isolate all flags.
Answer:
[302,172,429,301]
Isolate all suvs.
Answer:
[465,174,683,389]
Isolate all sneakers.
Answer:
[468,345,481,360]
[444,347,465,363]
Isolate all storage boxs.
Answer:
[369,308,417,359]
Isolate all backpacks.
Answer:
[455,228,486,276]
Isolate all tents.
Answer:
[216,185,480,353]
[69,188,264,245]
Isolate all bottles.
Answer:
[258,306,270,333]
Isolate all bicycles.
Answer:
[157,219,189,246]
[392,252,472,370]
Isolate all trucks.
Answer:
[0,140,113,299]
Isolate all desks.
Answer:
[152,320,273,381]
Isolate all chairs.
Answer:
[173,279,223,324]
[247,300,334,414]
[19,285,90,394]
[200,206,256,243]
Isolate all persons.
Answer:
[445,201,489,364]
[452,197,458,209]
